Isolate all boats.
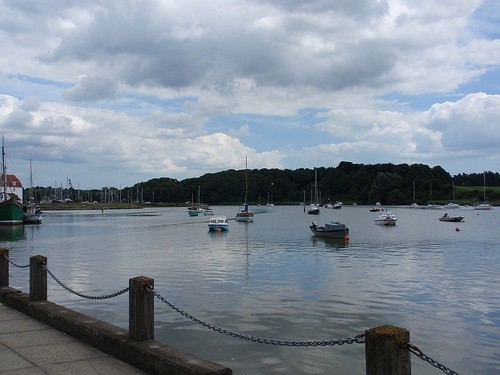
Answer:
[326,204,342,209]
[370,208,380,212]
[207,216,229,230]
[308,205,319,214]
[439,213,464,222]
[475,203,493,210]
[446,204,462,209]
[374,212,397,226]
[410,202,445,209]
[0,133,24,223]
[205,210,215,215]
[189,211,198,216]
[310,222,350,238]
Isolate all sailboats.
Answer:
[309,169,325,207]
[52,180,75,202]
[189,186,209,213]
[234,157,254,222]
[259,191,275,206]
[25,158,44,223]
